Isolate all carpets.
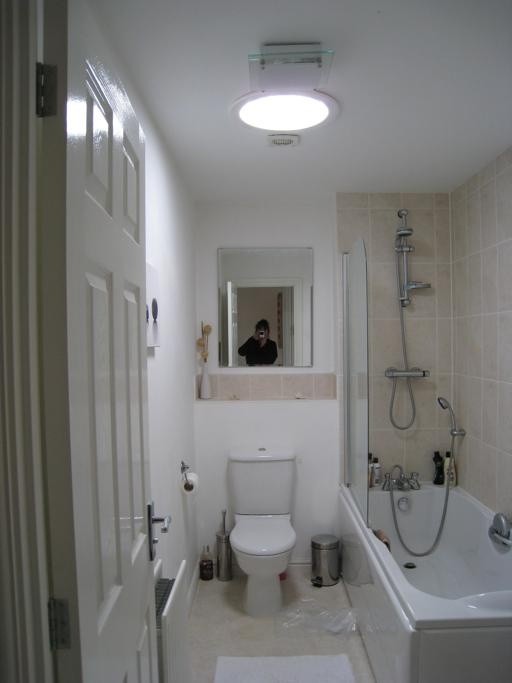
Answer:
[214,653,357,683]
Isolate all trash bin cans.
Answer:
[311,534,339,587]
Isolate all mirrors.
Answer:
[218,246,314,364]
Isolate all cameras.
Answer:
[259,331,265,338]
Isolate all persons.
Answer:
[238,319,278,366]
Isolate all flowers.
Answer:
[197,325,211,362]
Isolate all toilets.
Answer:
[224,447,297,616]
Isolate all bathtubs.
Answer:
[336,481,512,683]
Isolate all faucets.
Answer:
[397,473,410,491]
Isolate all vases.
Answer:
[200,363,212,398]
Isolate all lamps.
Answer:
[229,90,341,134]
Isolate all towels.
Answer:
[375,529,391,552]
